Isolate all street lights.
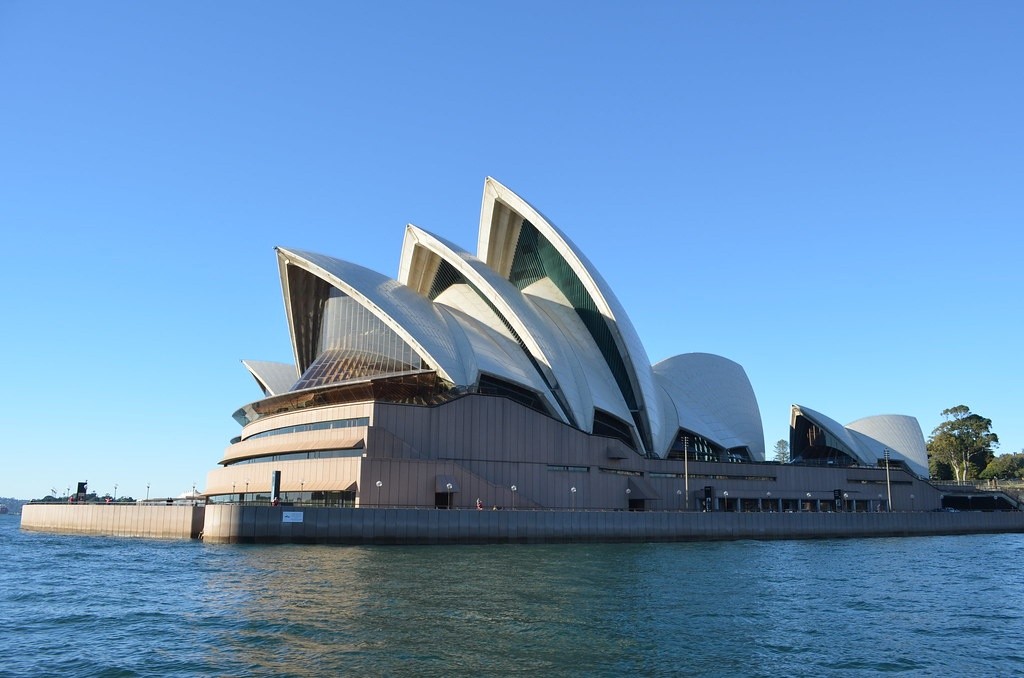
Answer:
[766,490,772,512]
[300,483,304,502]
[882,448,894,510]
[681,435,692,511]
[231,481,236,501]
[571,486,577,509]
[112,483,119,504]
[723,490,728,510]
[676,490,681,510]
[192,483,196,503]
[806,491,811,511]
[145,482,150,502]
[246,482,248,501]
[510,484,517,509]
[625,487,632,509]
[447,483,453,509]
[841,491,1004,510]
[376,480,383,507]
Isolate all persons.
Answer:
[166,498,173,506]
[105,496,112,504]
[476,497,483,510]
[272,497,278,506]
[68,495,75,504]
